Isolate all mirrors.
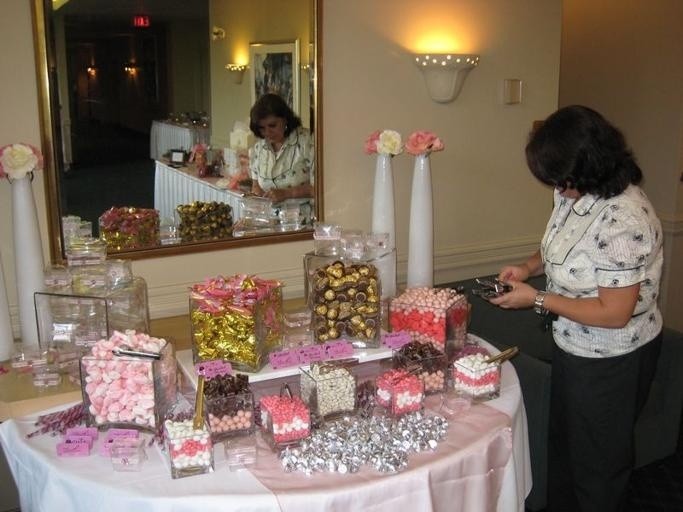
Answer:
[33,0,323,267]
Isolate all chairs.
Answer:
[437,275,683,512]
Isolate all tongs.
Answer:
[479,346,519,364]
[394,367,422,386]
[468,279,508,297]
[319,357,358,374]
[191,373,204,430]
[279,382,294,402]
[110,344,159,360]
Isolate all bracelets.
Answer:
[534,290,549,320]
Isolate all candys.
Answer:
[82,328,356,468]
[376,286,500,415]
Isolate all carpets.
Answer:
[61,162,85,183]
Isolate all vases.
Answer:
[4,171,55,353]
[369,154,397,301]
[406,154,433,290]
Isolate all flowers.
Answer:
[0,144,42,184]
[359,126,403,158]
[400,129,445,158]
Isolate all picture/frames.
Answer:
[246,39,300,122]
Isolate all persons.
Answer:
[248,92,314,226]
[489,105,664,511]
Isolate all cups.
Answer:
[258,401,310,452]
[373,379,423,415]
[108,437,145,472]
[388,295,471,356]
[452,361,500,403]
[185,287,284,371]
[303,248,394,349]
[298,365,357,418]
[227,438,257,470]
[309,219,392,260]
[59,197,313,263]
[390,346,448,393]
[102,258,133,286]
[42,260,74,291]
[27,276,152,370]
[202,392,253,439]
[162,420,213,478]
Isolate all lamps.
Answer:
[415,52,476,102]
[224,62,247,85]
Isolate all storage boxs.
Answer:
[186,272,288,372]
[97,206,159,255]
[382,285,472,364]
